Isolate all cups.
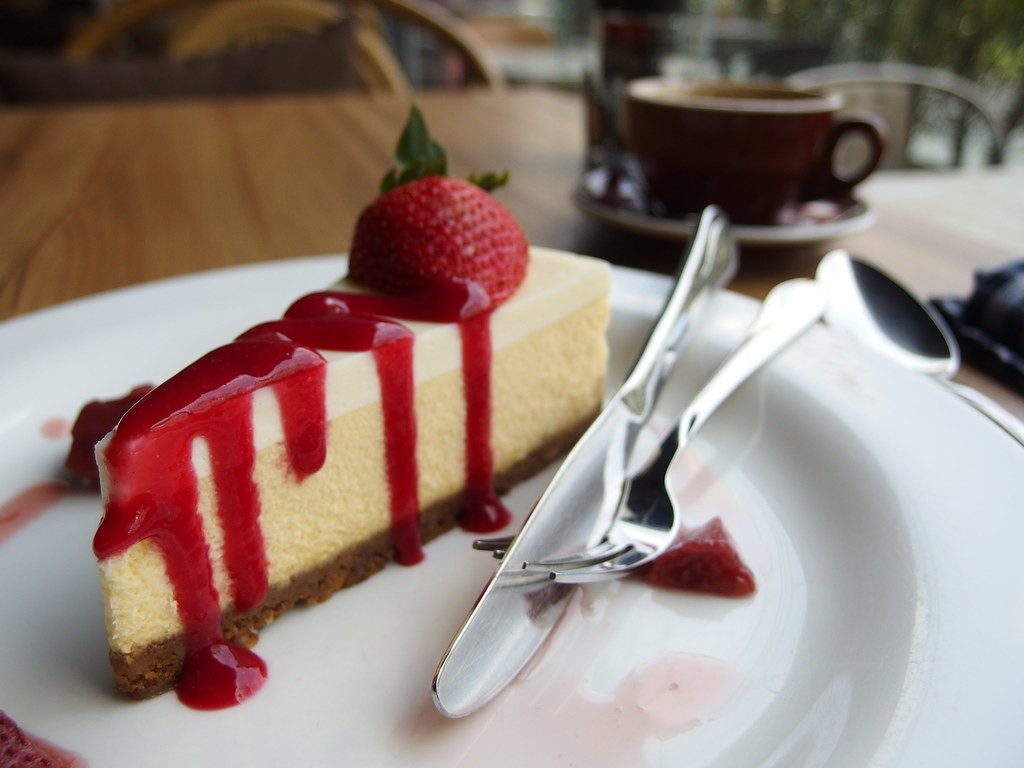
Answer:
[627,75,889,224]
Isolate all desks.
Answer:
[0,98,1024,432]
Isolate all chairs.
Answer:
[39,0,506,97]
[783,60,1004,171]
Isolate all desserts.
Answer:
[93,104,616,706]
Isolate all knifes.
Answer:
[432,205,740,717]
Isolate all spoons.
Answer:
[813,247,1024,451]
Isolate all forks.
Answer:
[472,280,829,583]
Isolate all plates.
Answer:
[573,166,882,255]
[1,252,1024,768]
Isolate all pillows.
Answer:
[12,13,360,103]
